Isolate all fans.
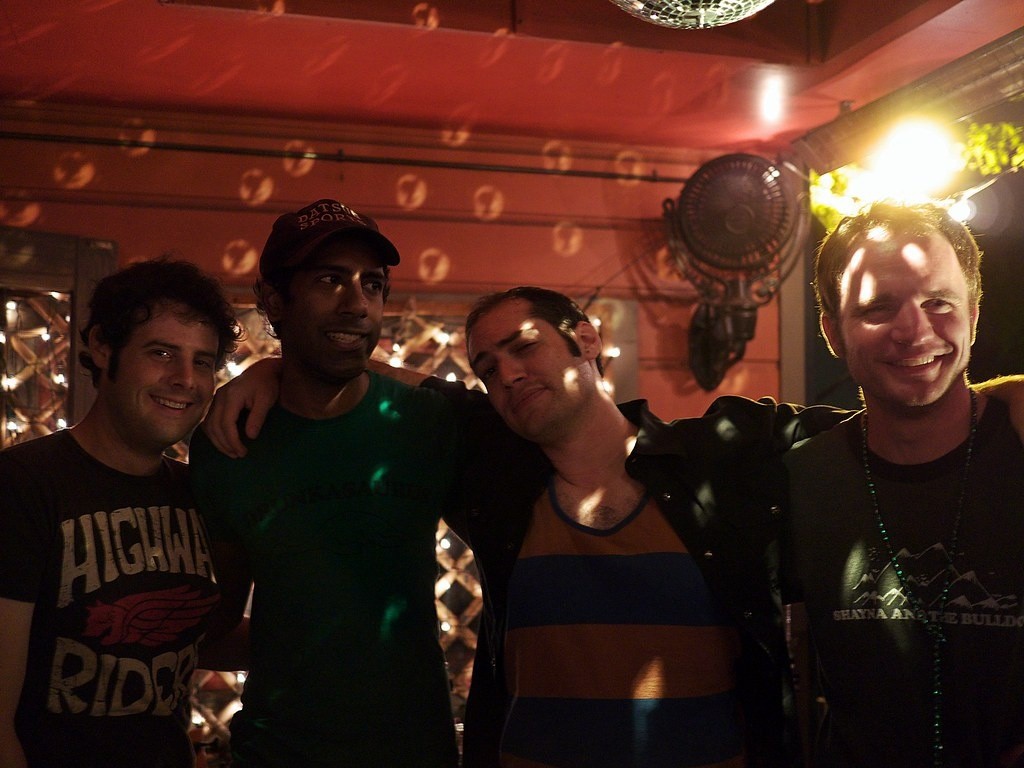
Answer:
[660,153,809,391]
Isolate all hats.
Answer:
[260,199,400,275]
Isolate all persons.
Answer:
[777,204,1024,767]
[206,286,1024,768]
[0,259,252,768]
[188,199,474,768]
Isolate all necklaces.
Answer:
[862,389,977,767]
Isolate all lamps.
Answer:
[613,0,773,29]
